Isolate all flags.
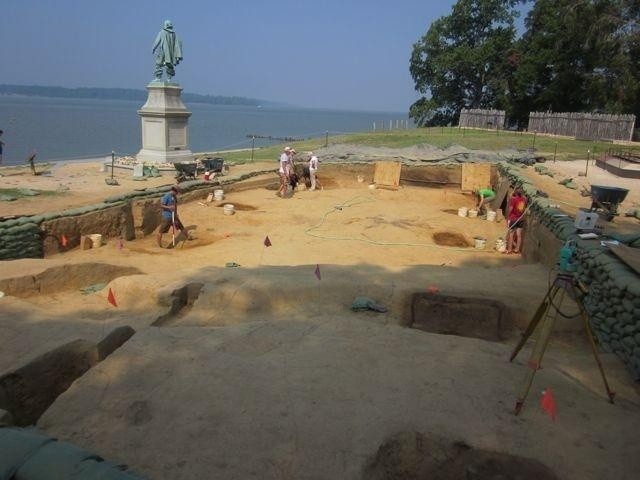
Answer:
[541,386,561,421]
[107,287,118,308]
[314,264,323,280]
[264,235,271,248]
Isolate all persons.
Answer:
[471,186,496,216]
[502,191,527,255]
[0,130,7,176]
[274,146,323,198]
[152,19,184,83]
[155,185,197,248]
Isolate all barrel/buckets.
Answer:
[458,207,468,218]
[474,239,486,250]
[224,203,234,216]
[214,189,224,200]
[487,211,497,221]
[88,234,102,247]
[469,210,479,217]
[496,239,506,252]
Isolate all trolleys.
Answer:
[174,161,198,183]
[201,158,224,173]
[582,182,629,222]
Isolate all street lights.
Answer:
[111,150,115,178]
[252,136,255,162]
[326,129,328,146]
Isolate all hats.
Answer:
[171,186,181,195]
[285,146,296,153]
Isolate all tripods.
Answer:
[508,273,616,415]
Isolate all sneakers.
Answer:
[503,250,519,254]
[187,235,194,240]
